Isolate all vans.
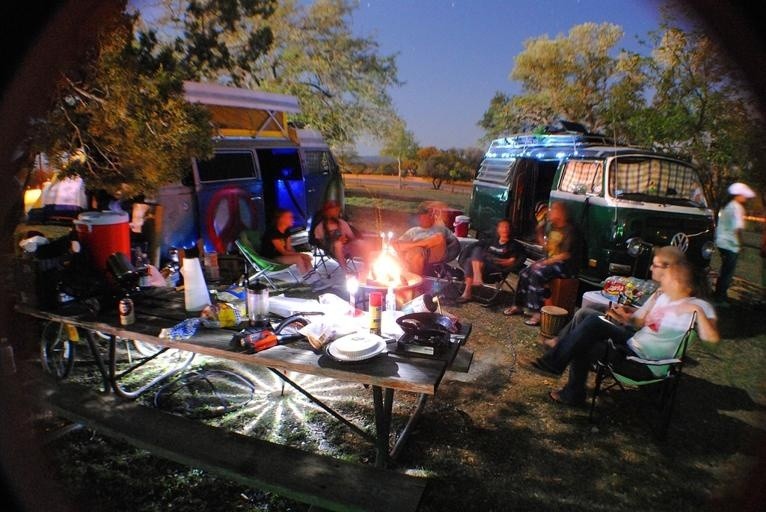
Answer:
[467,120,717,310]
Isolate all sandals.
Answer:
[502,305,524,316]
[548,390,579,406]
[391,240,405,256]
[516,358,561,379]
[523,313,541,326]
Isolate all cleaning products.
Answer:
[369,292,382,337]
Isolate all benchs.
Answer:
[1,356,429,511]
[443,343,474,373]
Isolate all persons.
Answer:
[314,200,371,282]
[390,207,447,276]
[260,208,334,293]
[518,261,720,406]
[503,201,584,327]
[713,182,757,308]
[534,245,687,351]
[455,219,520,304]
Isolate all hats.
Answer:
[727,182,757,198]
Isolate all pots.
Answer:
[398,311,464,340]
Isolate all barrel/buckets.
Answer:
[453,215,471,238]
[23,189,42,213]
[72,211,131,264]
[13,221,75,311]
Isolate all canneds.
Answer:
[118,298,135,326]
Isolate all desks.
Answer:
[7,279,474,471]
[582,289,640,312]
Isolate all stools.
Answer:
[550,276,581,319]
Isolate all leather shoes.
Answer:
[470,280,484,287]
[455,296,473,304]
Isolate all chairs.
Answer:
[585,310,702,425]
[425,234,530,309]
[306,208,364,280]
[235,229,302,290]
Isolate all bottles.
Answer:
[168,246,180,281]
[162,260,176,288]
[368,292,384,339]
[138,251,151,289]
[245,276,269,326]
[401,292,446,318]
[180,246,213,318]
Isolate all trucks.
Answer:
[26,80,346,284]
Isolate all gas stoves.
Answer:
[398,330,450,358]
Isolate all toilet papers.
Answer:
[179,257,213,313]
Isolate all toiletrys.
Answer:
[402,293,442,316]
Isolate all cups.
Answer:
[606,275,657,295]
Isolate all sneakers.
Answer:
[312,279,333,291]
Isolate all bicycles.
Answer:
[21,226,161,382]
